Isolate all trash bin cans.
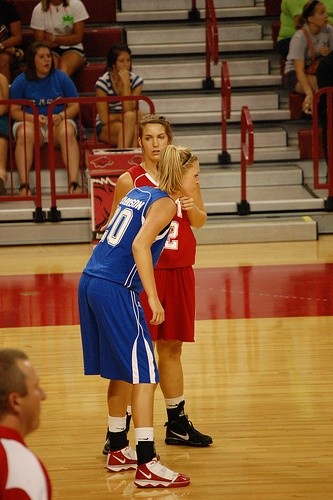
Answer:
[88,148,142,243]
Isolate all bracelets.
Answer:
[48,34,56,42]
[59,114,63,121]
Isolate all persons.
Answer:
[0,348,52,500]
[29,0,89,76]
[0,0,24,83]
[278,0,333,182]
[9,42,81,195]
[103,113,212,455]
[0,73,9,195]
[78,145,200,489]
[94,46,144,148]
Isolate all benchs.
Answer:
[6,0,131,171]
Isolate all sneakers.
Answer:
[102,439,129,455]
[134,457,190,488]
[106,446,160,472]
[163,414,213,446]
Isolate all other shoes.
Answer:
[19,184,31,196]
[69,183,81,194]
